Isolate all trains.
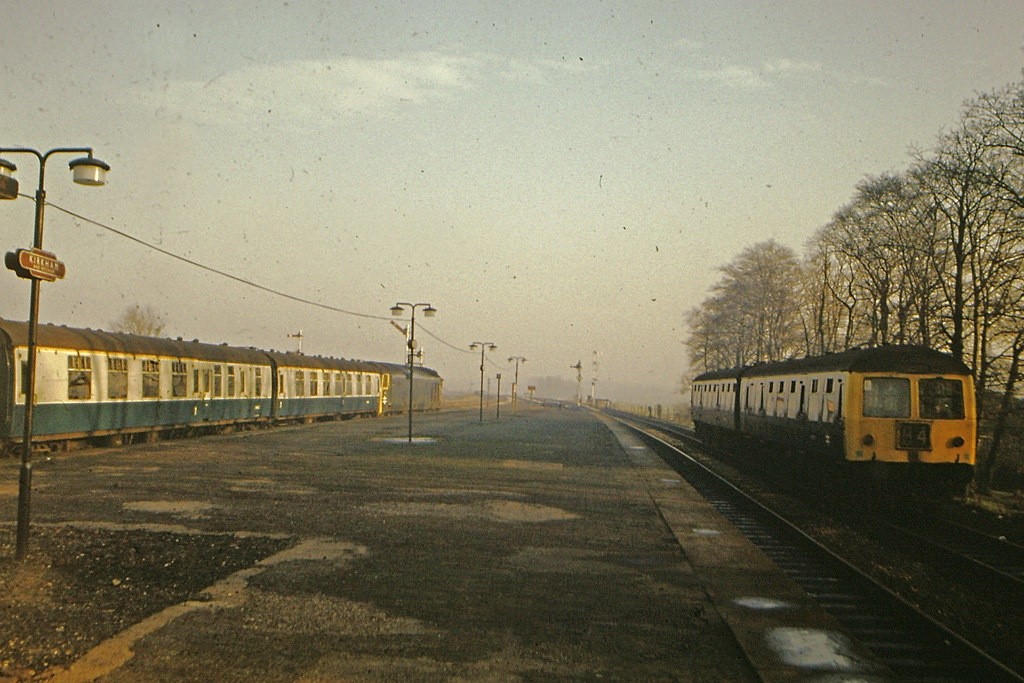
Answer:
[0,316,445,461]
[689,342,979,523]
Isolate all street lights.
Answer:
[469,341,498,422]
[0,146,111,563]
[508,356,527,415]
[389,302,437,443]
